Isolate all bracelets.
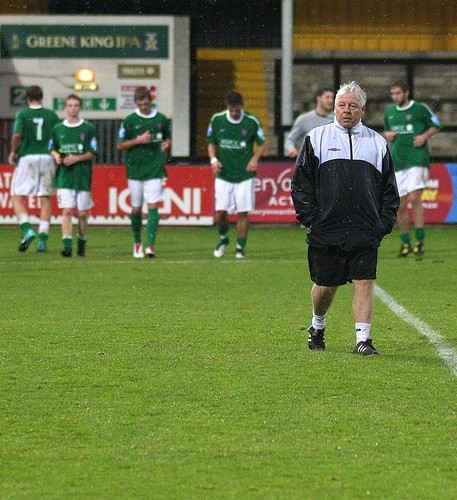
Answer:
[210,158,218,164]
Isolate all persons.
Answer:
[49,94,98,257]
[116,90,172,258]
[285,87,335,242]
[9,86,61,252]
[206,92,266,258]
[383,78,443,257]
[291,79,400,355]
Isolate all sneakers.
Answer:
[307,325,326,350]
[77,249,85,256]
[133,243,144,259]
[352,339,379,354]
[37,241,46,251]
[235,251,245,258]
[146,246,156,259]
[413,242,424,255]
[401,245,411,257]
[61,250,71,257]
[19,229,35,251]
[213,238,228,257]
